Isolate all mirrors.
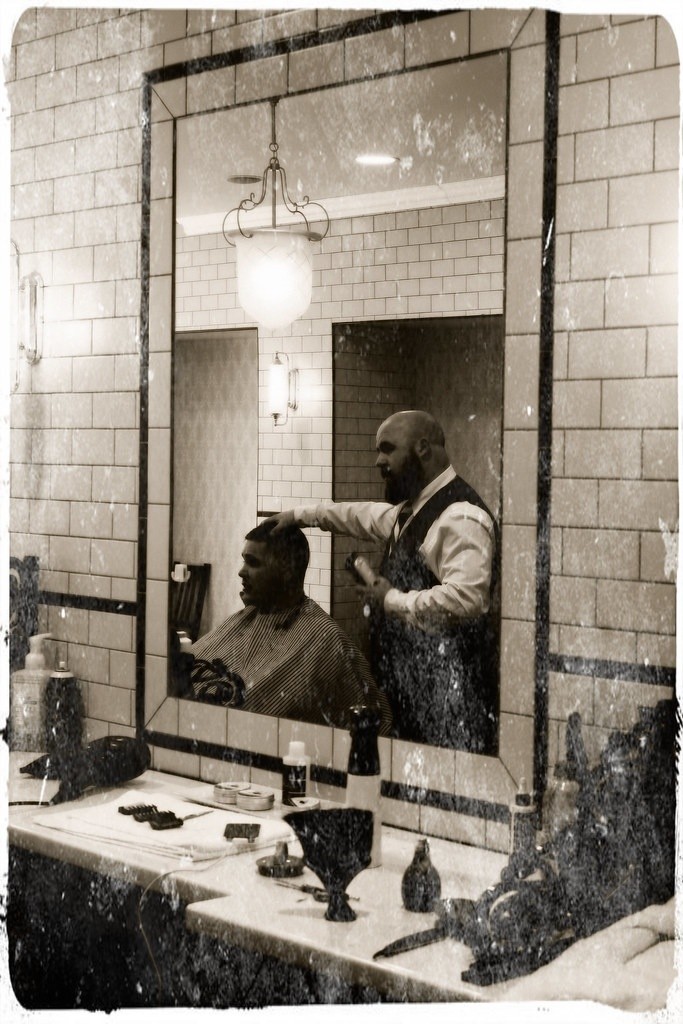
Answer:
[134,10,561,832]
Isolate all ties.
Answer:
[388,496,414,556]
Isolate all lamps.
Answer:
[267,350,296,426]
[222,99,330,330]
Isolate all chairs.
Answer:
[167,561,212,661]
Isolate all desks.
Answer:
[9,749,525,1002]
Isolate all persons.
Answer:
[187,521,393,738]
[262,411,499,756]
